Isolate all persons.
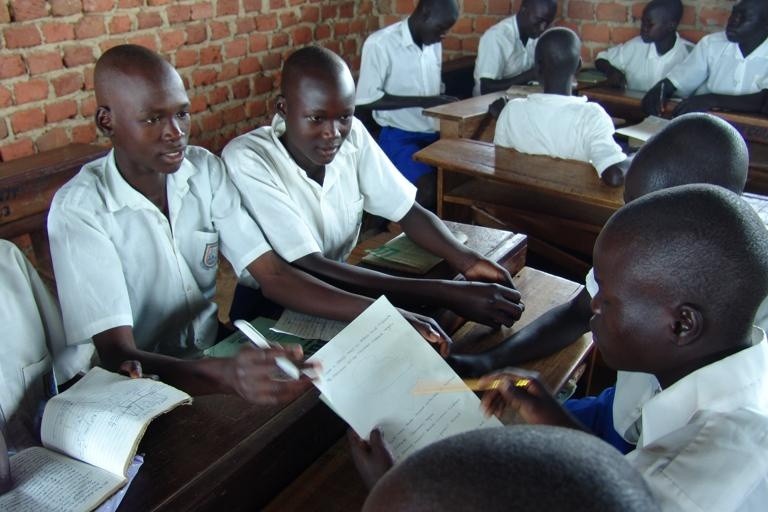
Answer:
[222,46,529,334]
[334,180,768,511]
[419,110,767,435]
[1,238,148,463]
[45,43,456,409]
[360,423,663,509]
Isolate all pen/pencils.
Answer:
[233,319,301,382]
[659,83,665,103]
[413,378,530,394]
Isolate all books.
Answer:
[198,316,328,372]
[360,229,471,276]
[0,364,194,512]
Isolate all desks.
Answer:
[111,220,597,511]
[411,67,767,279]
[2,141,112,304]
